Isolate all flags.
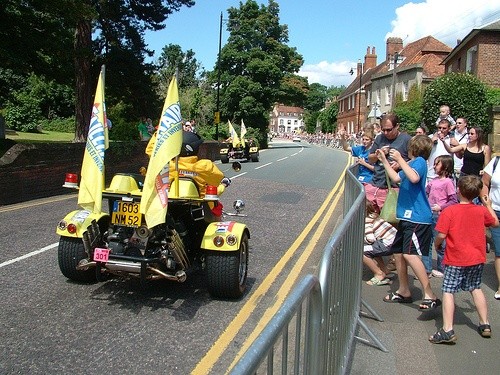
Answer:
[139,75,183,230]
[227,120,248,148]
[77,71,110,216]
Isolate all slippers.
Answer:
[383,290,412,303]
[366,276,390,286]
[418,298,441,312]
[385,273,395,278]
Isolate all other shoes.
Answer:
[413,272,432,280]
[387,259,396,271]
[432,270,444,278]
[494,291,500,298]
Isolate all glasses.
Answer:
[380,125,394,131]
[468,132,473,135]
[416,132,422,133]
[438,127,448,129]
[456,122,461,124]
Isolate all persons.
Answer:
[269,105,500,313]
[428,175,499,344]
[105,112,112,131]
[374,135,441,312]
[136,116,161,141]
[183,119,198,134]
[168,131,224,222]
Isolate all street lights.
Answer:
[213,11,241,141]
[349,65,362,135]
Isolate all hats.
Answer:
[179,131,204,157]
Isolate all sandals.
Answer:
[479,322,492,337]
[428,327,457,344]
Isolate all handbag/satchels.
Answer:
[478,181,491,203]
[379,189,401,224]
